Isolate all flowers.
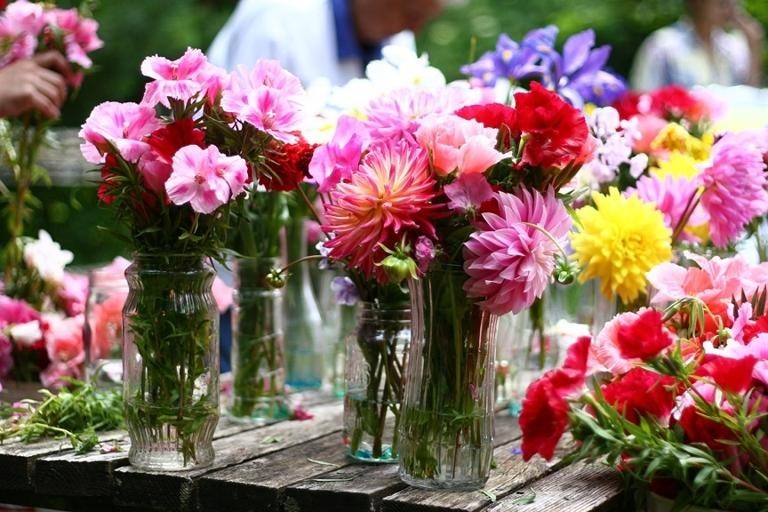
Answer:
[1,2,766,507]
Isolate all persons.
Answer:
[629,0,764,90]
[204,1,446,85]
[0,49,73,120]
